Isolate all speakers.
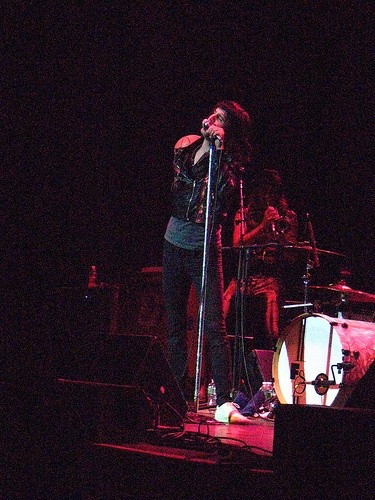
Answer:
[242,349,273,398]
[42,378,151,446]
[272,404,375,480]
[345,360,375,407]
[94,334,187,425]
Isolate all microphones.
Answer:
[268,205,275,231]
[202,119,224,143]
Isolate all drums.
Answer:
[271,312,375,408]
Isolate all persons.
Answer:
[220,169,298,341]
[158,100,250,419]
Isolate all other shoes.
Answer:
[214,402,248,423]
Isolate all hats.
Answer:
[252,168,283,187]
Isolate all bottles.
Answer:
[207,379,217,407]
[88,266,96,287]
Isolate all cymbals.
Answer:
[298,284,375,304]
[280,245,350,260]
